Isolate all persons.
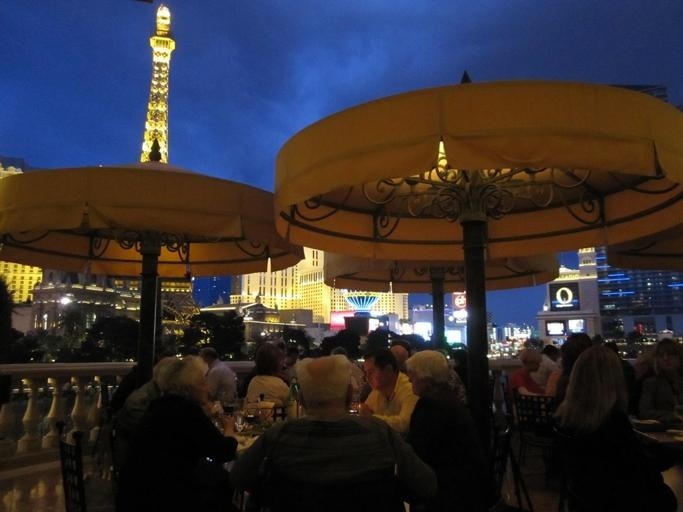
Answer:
[108,330,492,512]
[509,332,681,512]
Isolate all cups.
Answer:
[221,390,237,415]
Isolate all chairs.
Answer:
[56,382,120,511]
[497,375,573,510]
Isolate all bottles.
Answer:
[287,378,299,421]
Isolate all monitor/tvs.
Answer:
[545,320,566,336]
[568,319,586,334]
